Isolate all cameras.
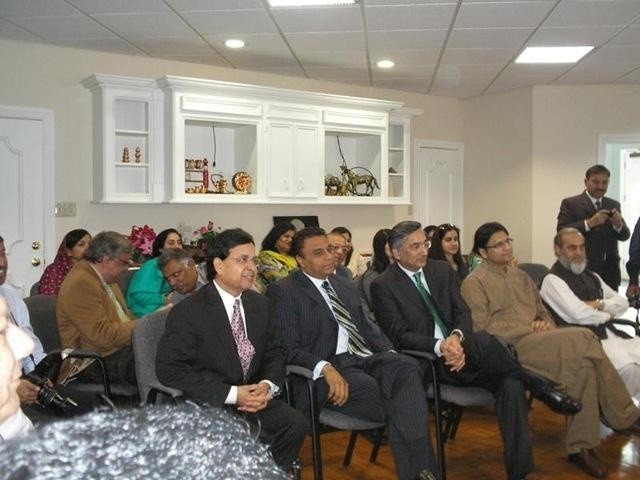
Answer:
[24,370,83,420]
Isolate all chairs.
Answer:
[28,279,42,296]
[286,349,448,480]
[517,262,640,341]
[402,332,536,442]
[21,294,138,402]
[131,305,323,479]
[115,265,141,301]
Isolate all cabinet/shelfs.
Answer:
[93,89,158,201]
[178,93,264,197]
[388,119,408,199]
[324,113,387,200]
[267,105,321,200]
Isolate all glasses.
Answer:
[390,241,432,251]
[221,257,258,265]
[483,239,514,249]
[437,224,455,239]
[331,245,349,254]
[112,257,131,266]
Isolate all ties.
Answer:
[596,201,606,252]
[198,281,207,289]
[323,281,373,356]
[232,301,256,377]
[107,286,133,322]
[414,272,448,340]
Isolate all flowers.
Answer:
[128,223,157,263]
[189,220,222,264]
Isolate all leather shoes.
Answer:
[363,430,389,445]
[531,383,582,413]
[416,470,435,480]
[569,448,609,476]
[612,411,639,435]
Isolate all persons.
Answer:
[325,232,353,281]
[540,228,640,401]
[127,229,196,318]
[39,228,93,295]
[367,220,584,475]
[360,229,394,306]
[430,224,468,283]
[264,224,438,476]
[56,231,141,386]
[331,226,353,270]
[0,294,34,429]
[423,226,437,251]
[555,166,630,297]
[1,237,57,424]
[255,221,299,288]
[157,245,218,305]
[460,221,640,474]
[625,214,640,308]
[154,227,307,476]
